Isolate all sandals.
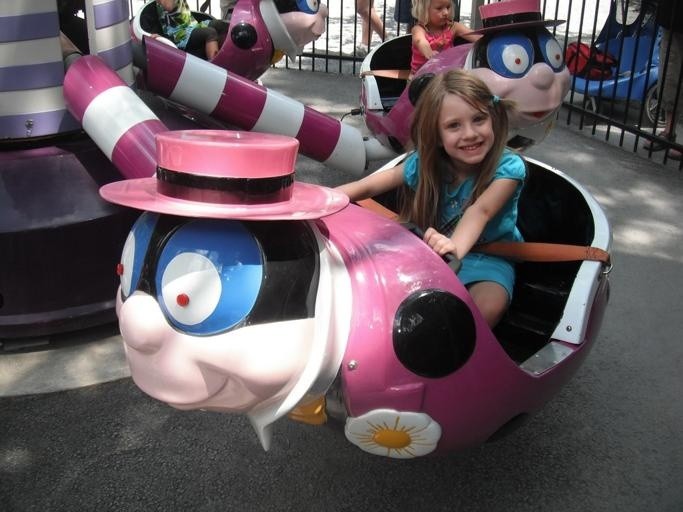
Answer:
[643,132,683,160]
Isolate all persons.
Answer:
[153,0,230,64]
[405,0,484,85]
[347,0,390,59]
[331,66,529,331]
[643,0,682,160]
